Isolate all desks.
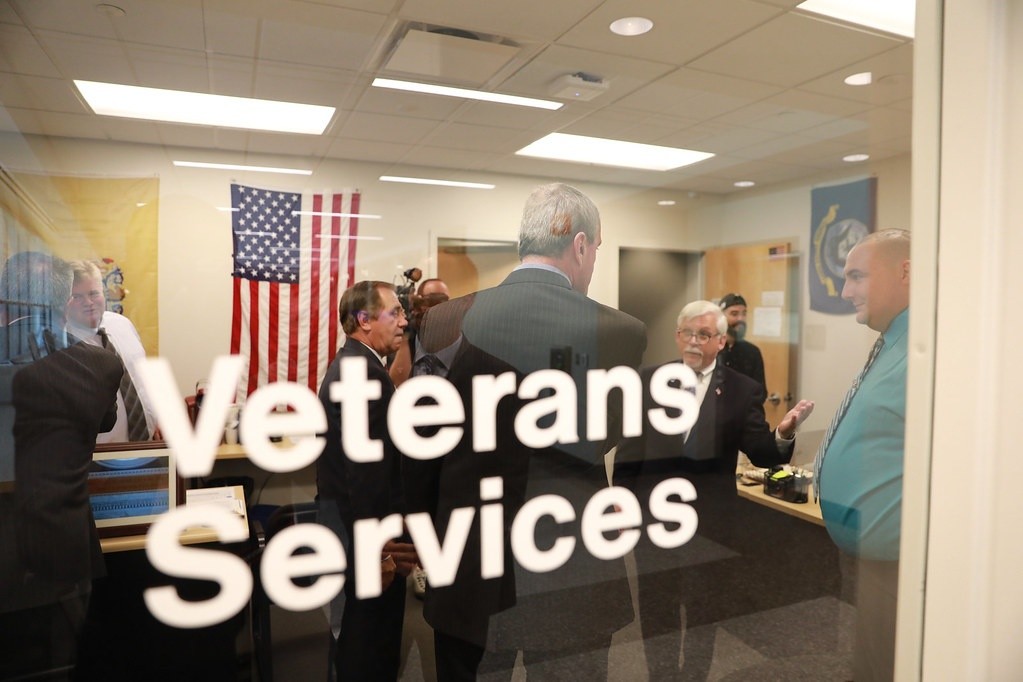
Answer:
[100,525,273,682]
[180,435,318,506]
[720,463,842,659]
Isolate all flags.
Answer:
[230,177,361,414]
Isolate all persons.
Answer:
[716,293,766,391]
[67,259,159,443]
[387,279,450,388]
[0,250,124,682]
[612,301,815,682]
[421,183,650,681]
[316,281,410,682]
[815,228,910,682]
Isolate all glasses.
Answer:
[678,329,720,344]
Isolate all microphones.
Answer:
[405,268,422,282]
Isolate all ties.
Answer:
[681,372,701,440]
[96,327,150,442]
[813,334,885,504]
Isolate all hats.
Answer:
[719,294,746,310]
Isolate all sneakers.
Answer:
[412,568,426,598]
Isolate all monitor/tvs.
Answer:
[87,448,176,538]
